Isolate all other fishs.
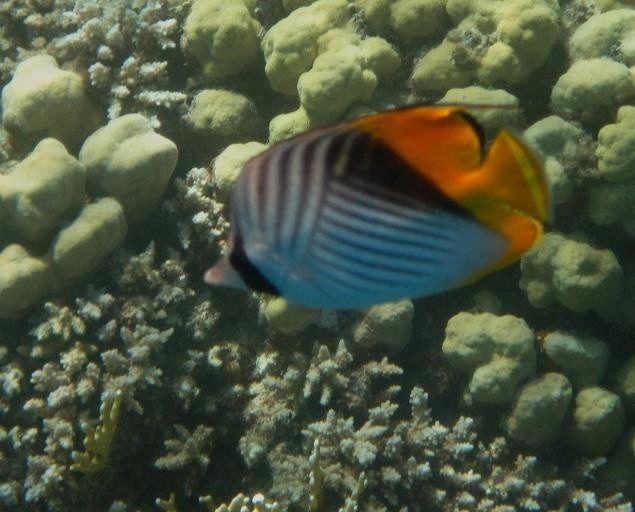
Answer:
[203,103,555,311]
